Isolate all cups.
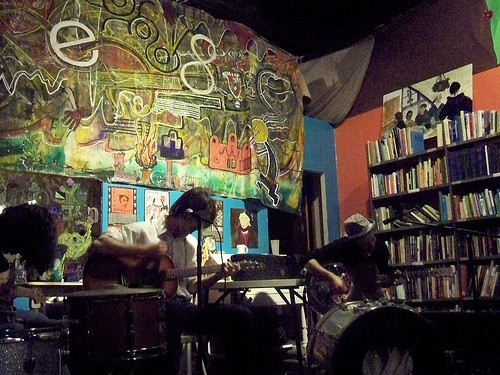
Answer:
[237,244,249,254]
[271,240,279,255]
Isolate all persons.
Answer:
[0,201,56,326]
[302,214,395,310]
[83,185,258,375]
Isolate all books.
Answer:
[363,110,500,302]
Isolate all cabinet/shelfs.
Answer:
[365,132,500,313]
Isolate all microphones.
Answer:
[383,208,416,224]
[185,208,214,224]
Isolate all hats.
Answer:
[344,213,375,238]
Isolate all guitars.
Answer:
[307,261,455,308]
[82,251,266,299]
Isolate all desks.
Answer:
[208,278,308,366]
[27,280,84,288]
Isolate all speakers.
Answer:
[0,332,64,375]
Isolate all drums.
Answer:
[63,286,168,366]
[0,323,65,375]
[306,298,446,375]
